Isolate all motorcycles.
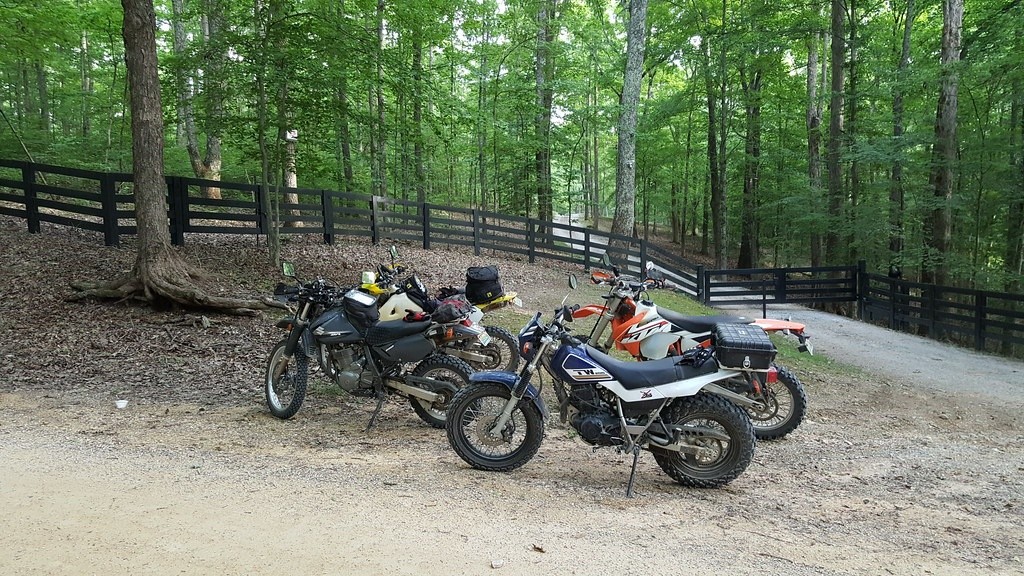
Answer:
[446,273,781,500]
[263,253,524,433]
[571,251,813,442]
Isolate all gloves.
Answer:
[403,309,430,323]
[674,346,715,368]
[439,286,458,299]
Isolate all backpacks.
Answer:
[466,266,504,305]
